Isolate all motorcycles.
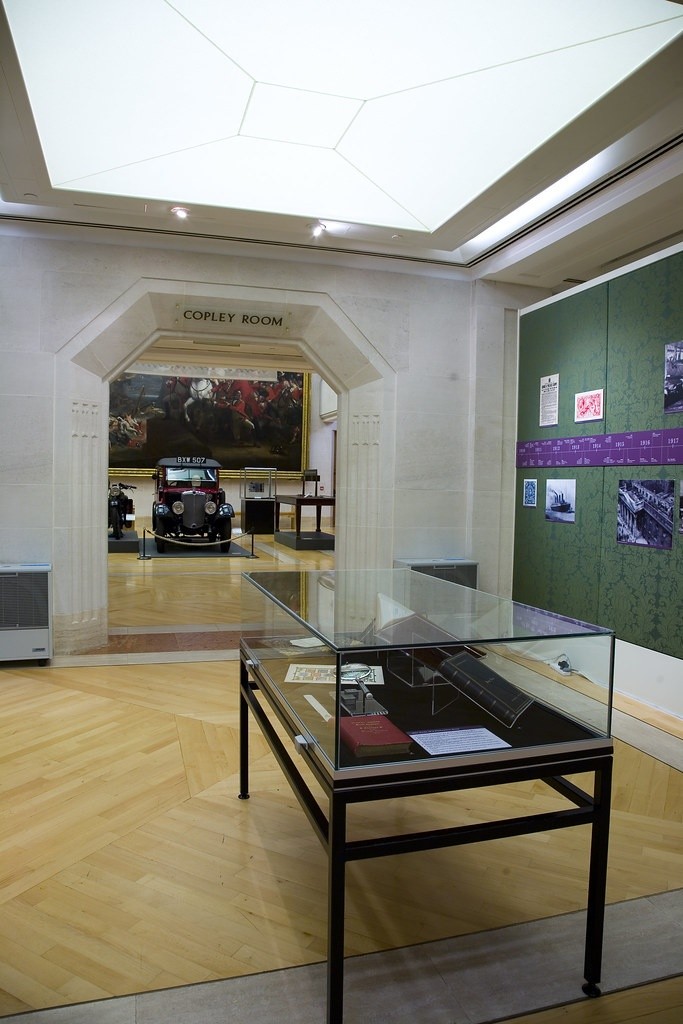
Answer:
[107,479,137,540]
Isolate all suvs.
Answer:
[152,456,235,553]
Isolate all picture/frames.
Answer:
[107,360,312,480]
[663,339,683,413]
[616,479,674,550]
[545,479,576,523]
[522,479,537,507]
[574,389,604,423]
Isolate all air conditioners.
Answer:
[394,557,478,618]
[1,562,54,668]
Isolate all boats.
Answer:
[549,492,571,511]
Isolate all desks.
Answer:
[241,497,281,535]
[238,629,615,1024]
[273,494,335,539]
